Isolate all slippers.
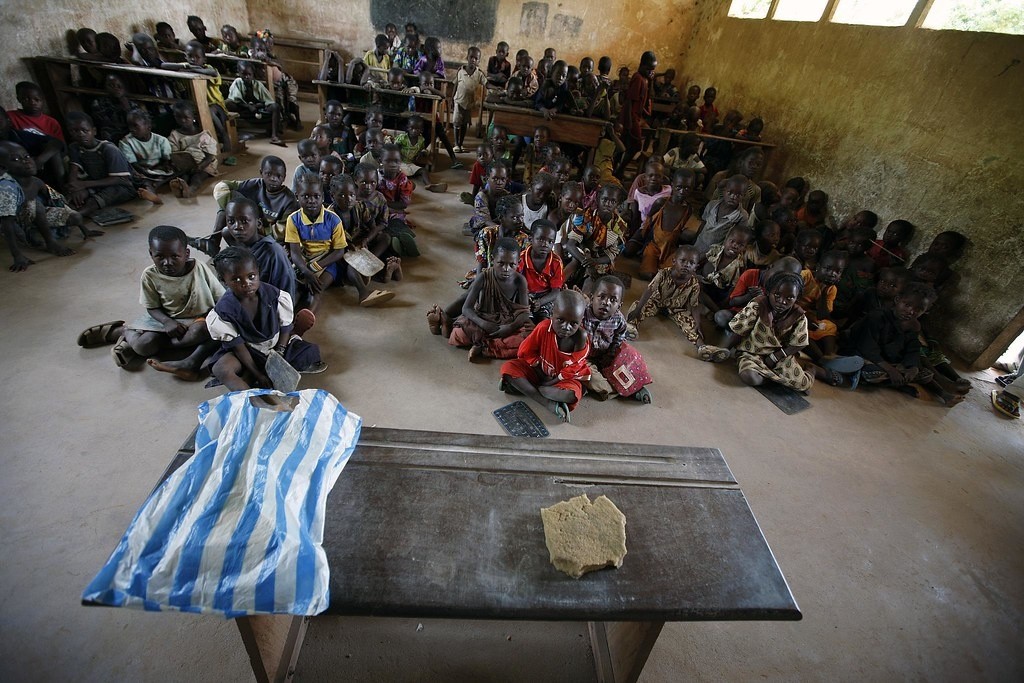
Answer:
[633,387,651,404]
[111,335,134,367]
[995,374,1017,386]
[361,289,395,306]
[77,321,125,346]
[556,403,570,423]
[992,390,1020,420]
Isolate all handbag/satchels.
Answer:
[603,341,651,398]
[81,389,362,619]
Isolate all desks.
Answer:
[40,55,222,173]
[160,47,276,132]
[483,100,611,170]
[311,79,445,158]
[660,127,776,161]
[249,31,337,102]
[83,424,803,683]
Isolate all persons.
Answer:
[0,105,70,189]
[0,139,106,274]
[206,247,329,413]
[166,98,220,198]
[7,80,72,163]
[116,108,179,204]
[76,225,228,369]
[75,15,762,152]
[56,111,137,216]
[424,285,1024,423]
[283,171,349,340]
[145,199,297,381]
[326,174,397,308]
[188,153,965,284]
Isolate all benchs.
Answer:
[66,87,246,160]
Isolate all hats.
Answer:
[255,29,273,51]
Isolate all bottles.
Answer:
[408,95,415,114]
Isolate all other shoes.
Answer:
[391,237,408,258]
[850,370,861,391]
[269,138,283,144]
[451,162,464,168]
[825,356,864,373]
[820,366,843,386]
[453,147,460,152]
[289,309,314,337]
[400,233,420,257]
[461,146,468,152]
[187,237,220,257]
[624,323,638,340]
[697,345,730,362]
[225,156,237,165]
[461,192,474,206]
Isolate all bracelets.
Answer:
[277,343,287,354]
[310,261,323,272]
[769,353,779,362]
[781,348,788,358]
